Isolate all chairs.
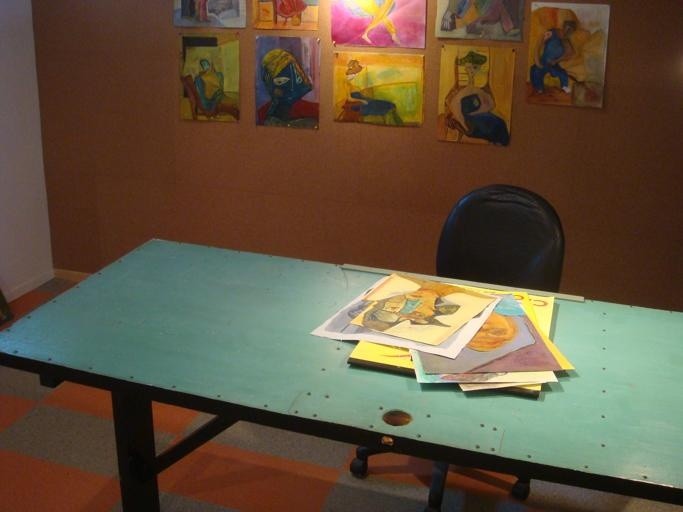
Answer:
[351,185,566,511]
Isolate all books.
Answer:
[347,284,556,400]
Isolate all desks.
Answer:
[0,236,683,511]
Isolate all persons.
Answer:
[448,49,511,148]
[528,19,579,96]
